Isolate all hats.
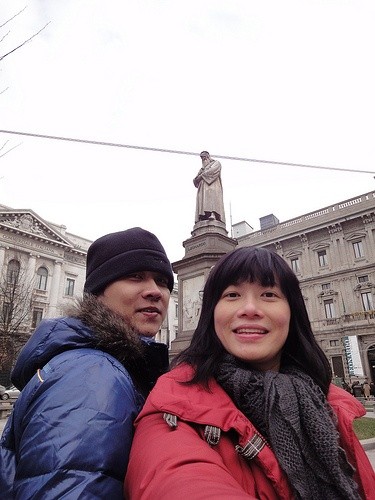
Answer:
[83,227,174,295]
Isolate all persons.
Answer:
[0,227,174,499]
[194,150,225,223]
[341,379,375,401]
[122,245,375,500]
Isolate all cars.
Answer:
[0,384,21,401]
